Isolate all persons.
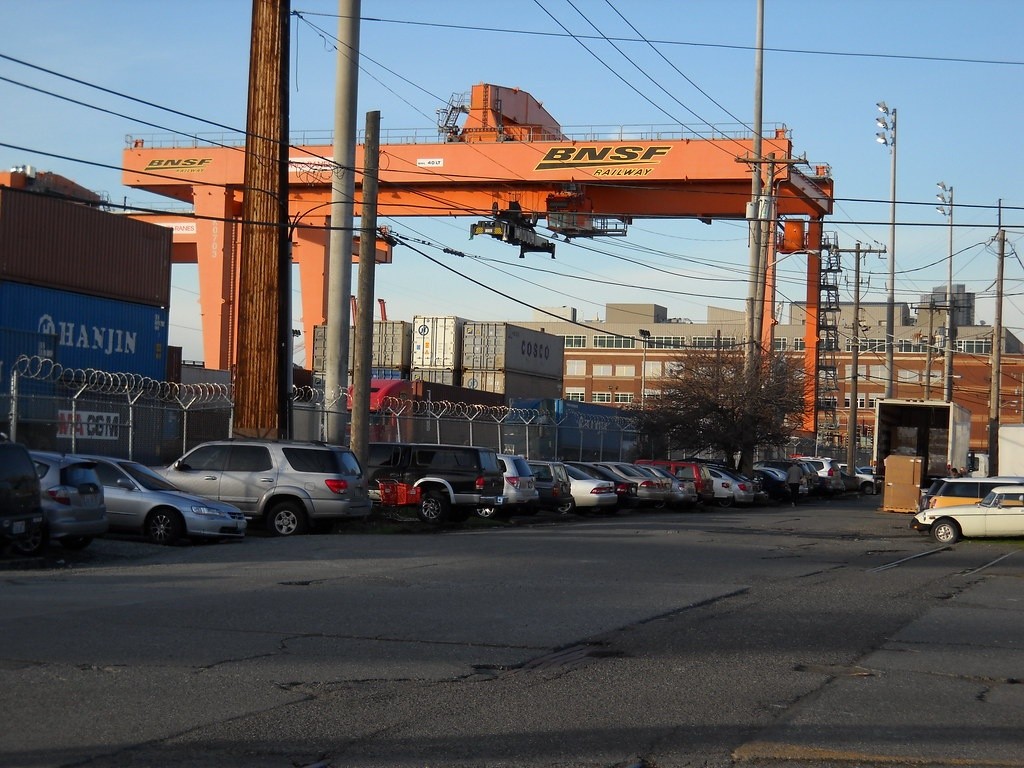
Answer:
[785,460,803,507]
[951,467,967,478]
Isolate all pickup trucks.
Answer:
[368,442,504,525]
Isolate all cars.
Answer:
[909,486,1024,545]
[554,463,618,514]
[62,453,247,546]
[562,457,881,509]
[0,432,43,558]
[28,450,110,549]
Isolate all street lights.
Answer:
[846,341,902,476]
[919,374,963,485]
[639,329,650,412]
[741,248,821,476]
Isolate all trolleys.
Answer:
[376,478,421,527]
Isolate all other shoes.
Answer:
[791,502,796,508]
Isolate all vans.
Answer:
[920,477,1024,511]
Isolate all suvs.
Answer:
[148,438,371,536]
[473,453,539,519]
[514,459,573,516]
